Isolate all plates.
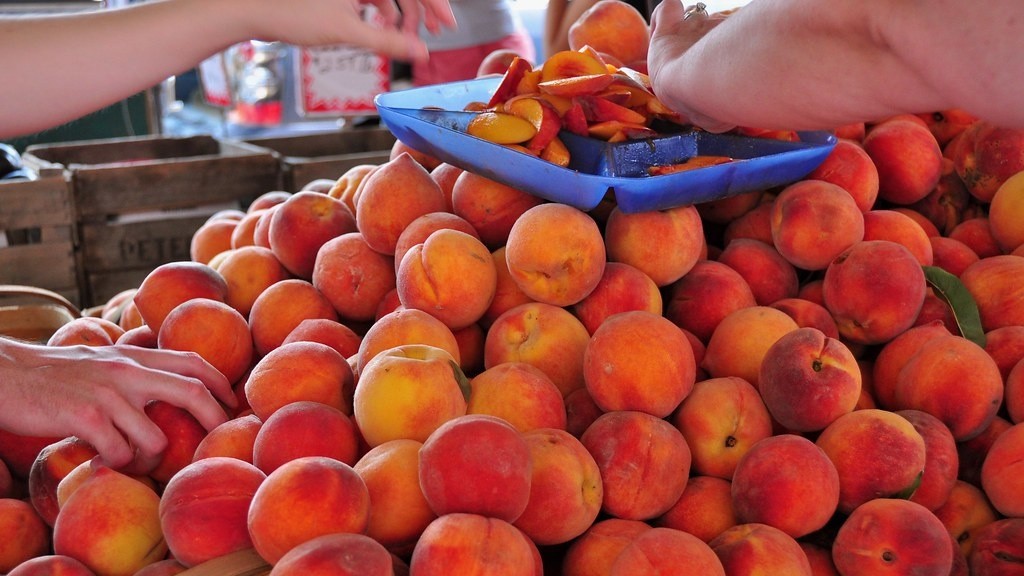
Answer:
[374,74,838,213]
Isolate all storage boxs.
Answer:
[225,128,398,194]
[22,133,283,305]
[0,157,87,312]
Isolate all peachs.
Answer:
[0,0,1024,576]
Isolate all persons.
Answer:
[0,0,1024,478]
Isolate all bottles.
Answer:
[239,40,282,107]
